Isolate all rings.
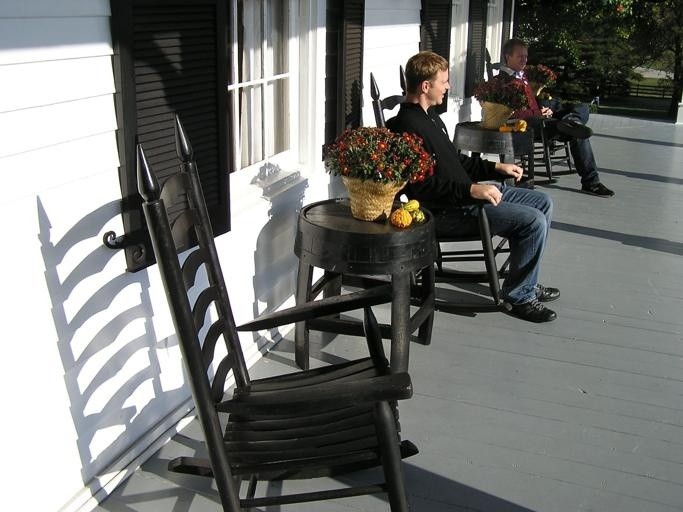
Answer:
[489,197,493,201]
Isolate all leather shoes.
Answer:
[502,299,557,323]
[535,283,560,302]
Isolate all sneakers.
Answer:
[581,182,615,198]
[557,118,593,139]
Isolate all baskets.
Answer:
[528,81,546,96]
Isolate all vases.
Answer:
[528,82,544,97]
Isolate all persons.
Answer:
[389,50,560,322]
[499,38,614,198]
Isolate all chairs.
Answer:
[370,64,513,312]
[485,48,577,184]
[136,115,419,512]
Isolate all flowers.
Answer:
[474,76,528,109]
[322,125,437,185]
[524,64,557,86]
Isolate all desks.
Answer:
[295,198,435,375]
[452,121,534,189]
